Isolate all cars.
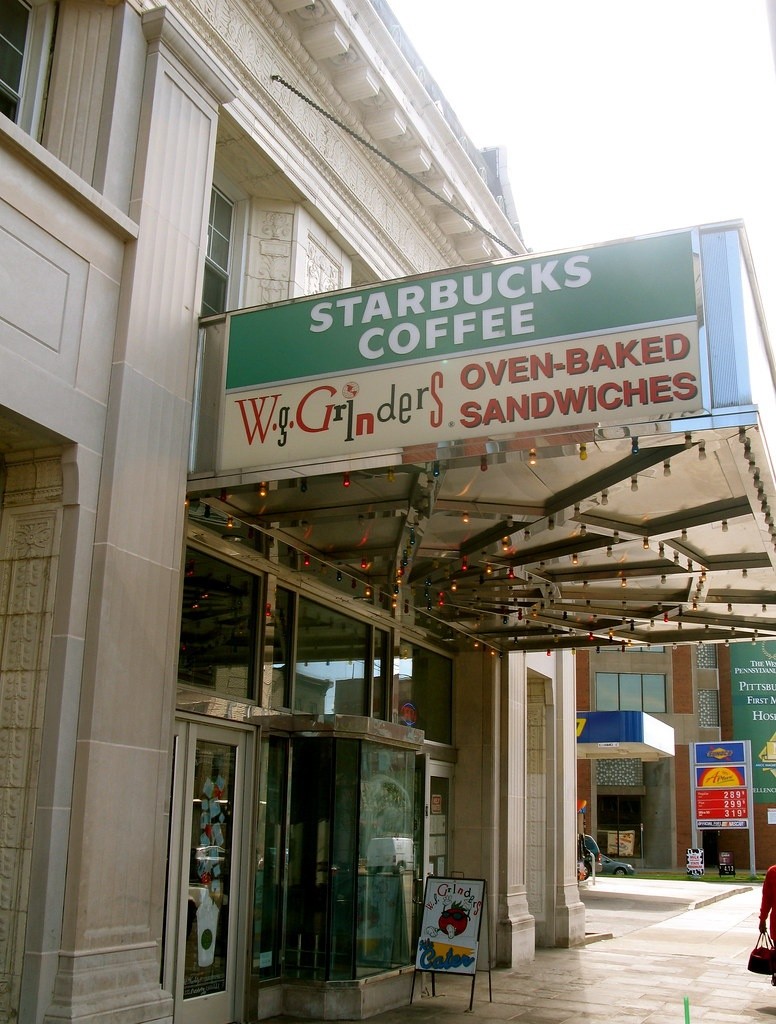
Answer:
[600,853,635,876]
[578,835,603,879]
[190,846,229,880]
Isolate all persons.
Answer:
[759,865,776,985]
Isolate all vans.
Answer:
[367,837,417,876]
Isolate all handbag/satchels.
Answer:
[748,930,776,975]
